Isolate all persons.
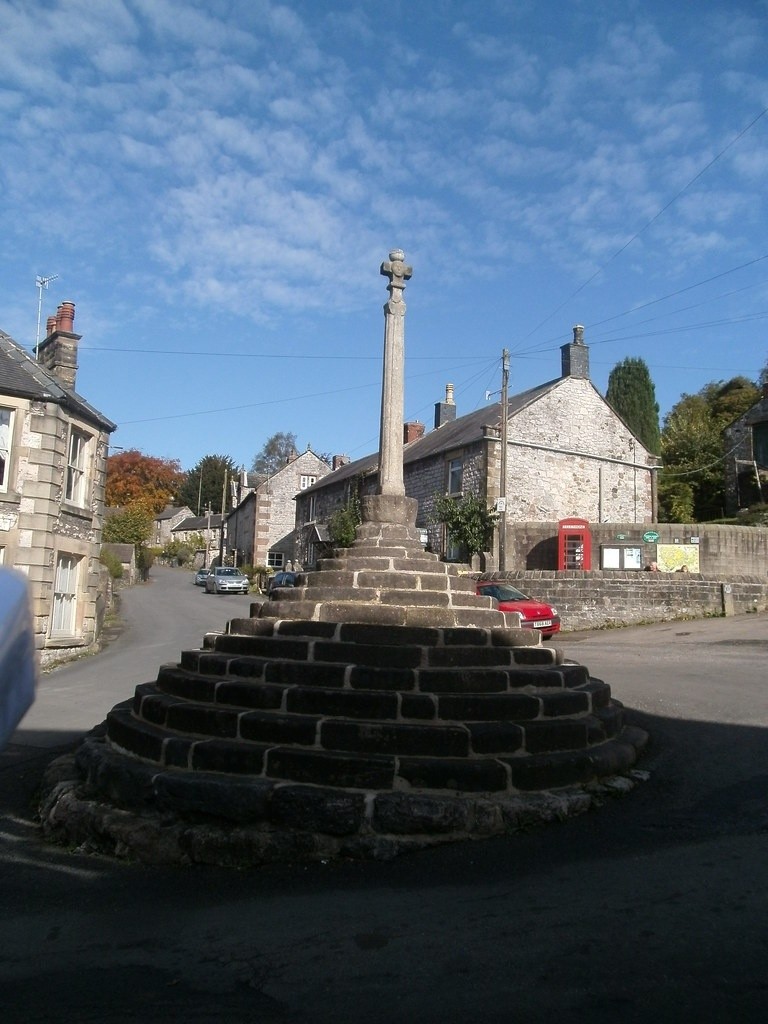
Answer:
[645,560,689,573]
[286,558,304,573]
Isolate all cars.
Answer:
[267,571,301,602]
[194,569,211,586]
[205,567,250,594]
[476,582,560,641]
